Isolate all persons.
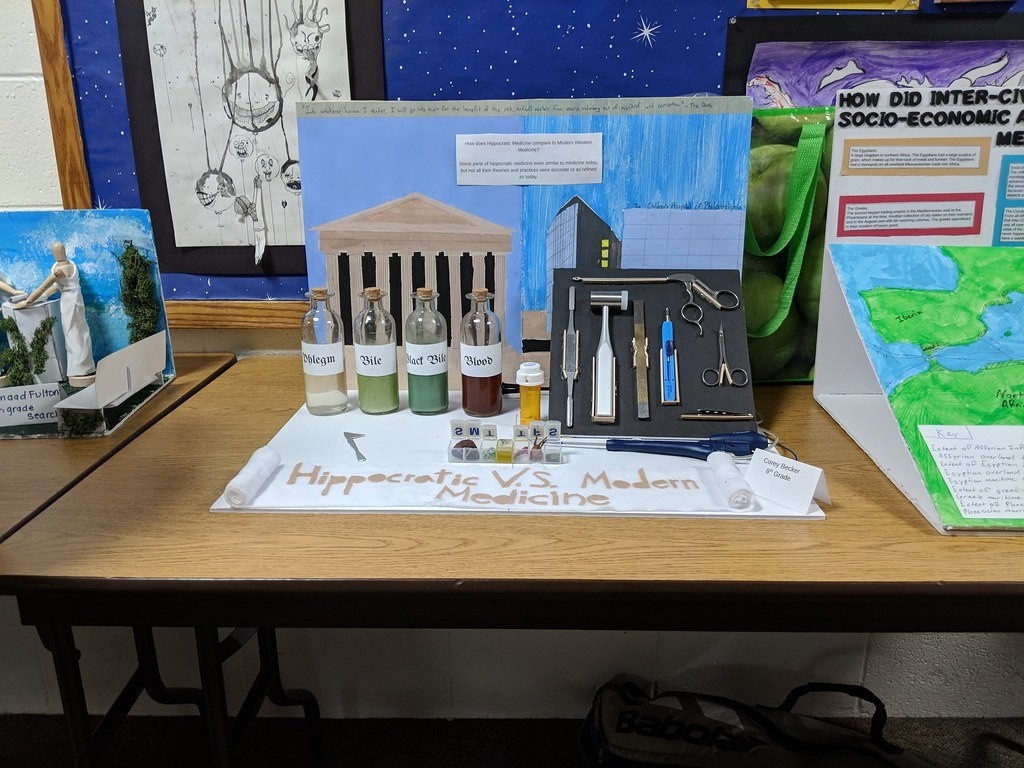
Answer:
[12,242,96,377]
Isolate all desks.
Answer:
[0,356,1022,767]
[0,353,237,542]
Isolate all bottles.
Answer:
[405,288,449,415]
[460,288,502,418]
[353,287,399,415]
[301,288,348,417]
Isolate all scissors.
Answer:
[572,271,739,337]
[700,322,749,388]
